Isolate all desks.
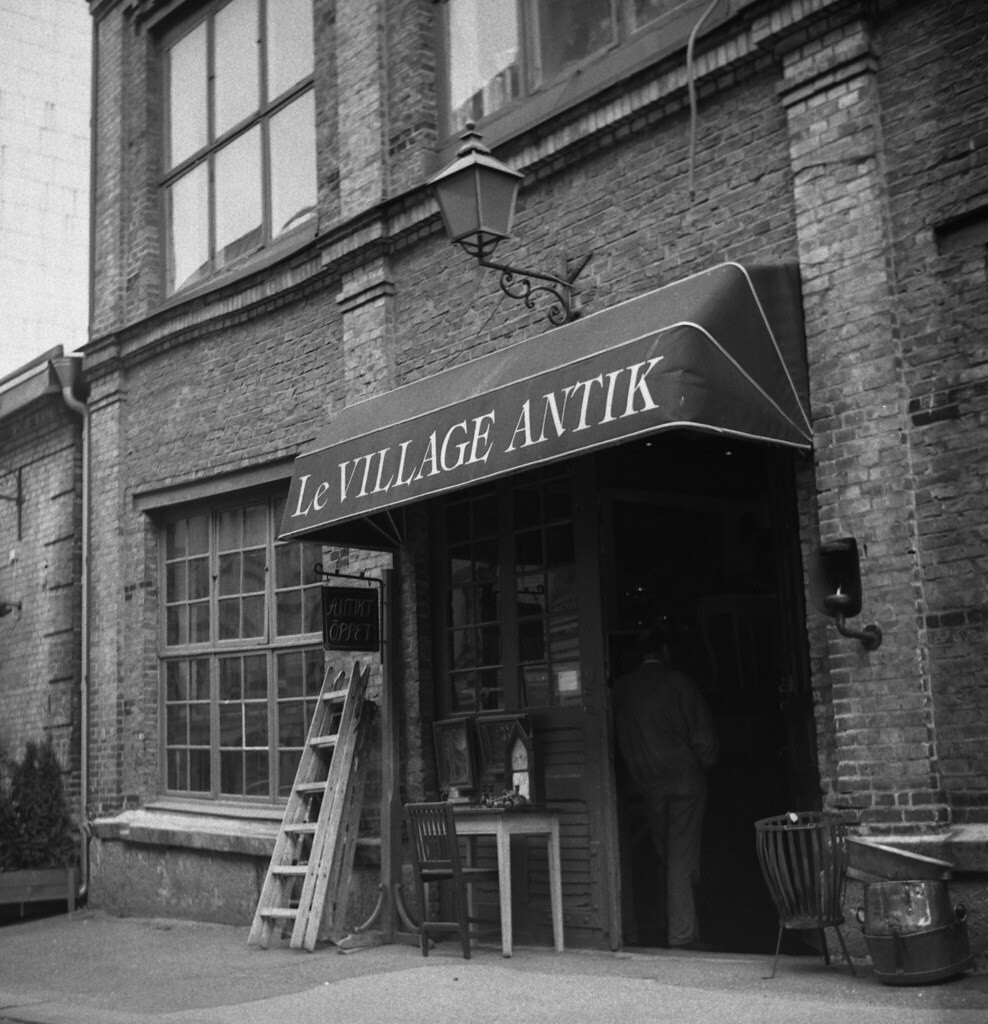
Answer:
[417,807,567,956]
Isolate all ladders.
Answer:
[245,658,380,952]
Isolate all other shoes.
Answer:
[680,937,712,953]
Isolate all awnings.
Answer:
[278,261,816,547]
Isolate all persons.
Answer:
[612,625,721,951]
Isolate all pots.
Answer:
[855,880,954,934]
[864,904,973,985]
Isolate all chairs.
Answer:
[403,802,500,960]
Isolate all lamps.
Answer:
[812,538,883,651]
[426,115,575,328]
